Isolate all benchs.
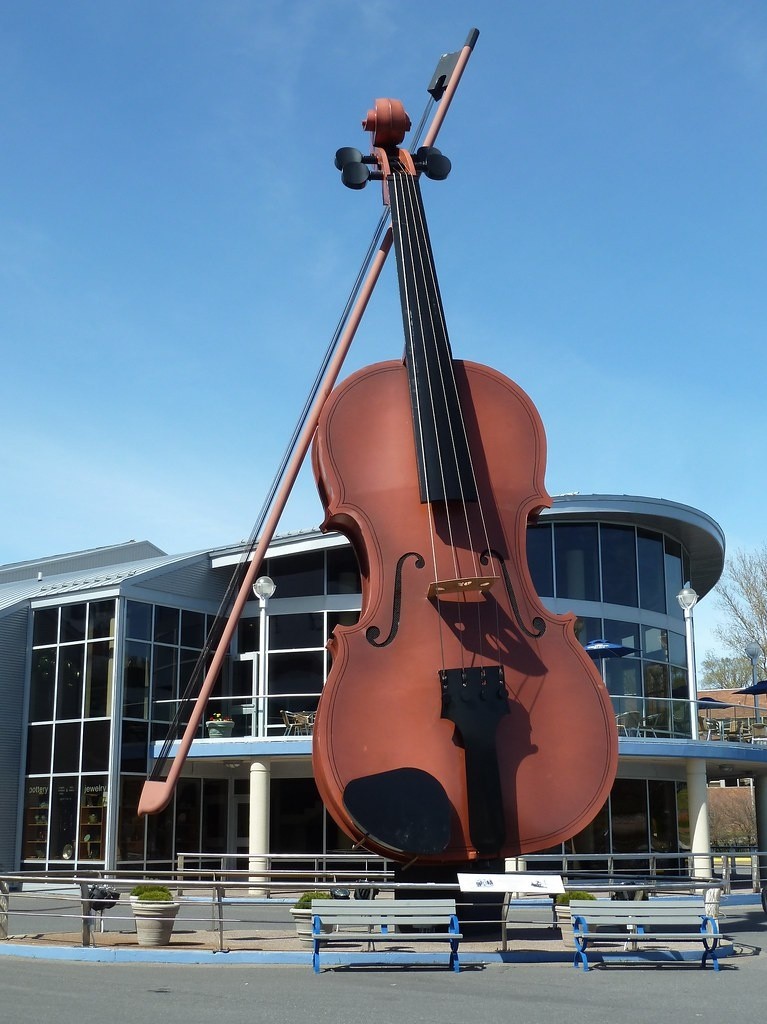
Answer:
[311,899,463,975]
[570,899,724,972]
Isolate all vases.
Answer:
[206,721,235,738]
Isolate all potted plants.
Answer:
[131,891,180,946]
[289,892,333,948]
[555,891,597,947]
[130,885,170,932]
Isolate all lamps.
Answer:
[330,888,350,900]
[89,884,110,911]
[611,881,649,901]
[354,881,380,900]
[105,888,121,909]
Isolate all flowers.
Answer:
[210,713,232,721]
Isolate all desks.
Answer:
[715,719,730,741]
[295,711,314,735]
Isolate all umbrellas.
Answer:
[698,697,734,709]
[731,680,767,695]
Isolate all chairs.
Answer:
[629,713,661,738]
[280,710,317,736]
[698,716,767,749]
[615,711,641,737]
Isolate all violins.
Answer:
[305,95,621,866]
